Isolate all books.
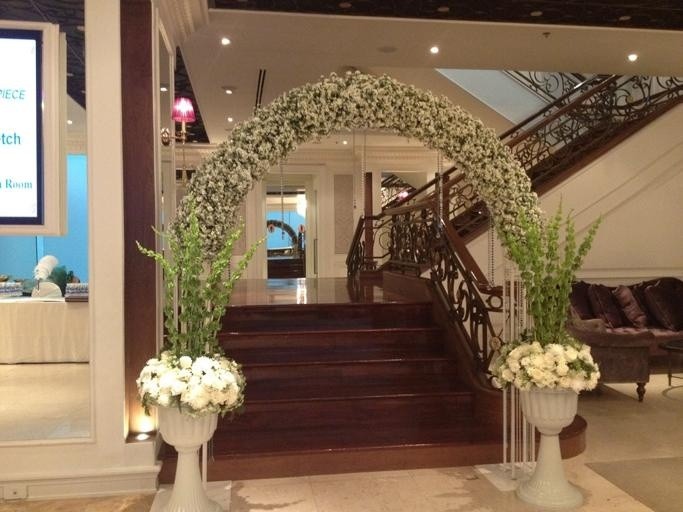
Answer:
[0,282,23,299]
[64,283,88,302]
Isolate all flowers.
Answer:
[165,68,551,261]
[131,190,266,420]
[485,189,604,397]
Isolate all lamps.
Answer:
[160,94,198,146]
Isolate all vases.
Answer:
[508,378,587,510]
[145,401,224,511]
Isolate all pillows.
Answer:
[612,284,647,328]
[587,282,619,326]
[641,278,680,332]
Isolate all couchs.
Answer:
[567,317,654,403]
[557,276,683,402]
